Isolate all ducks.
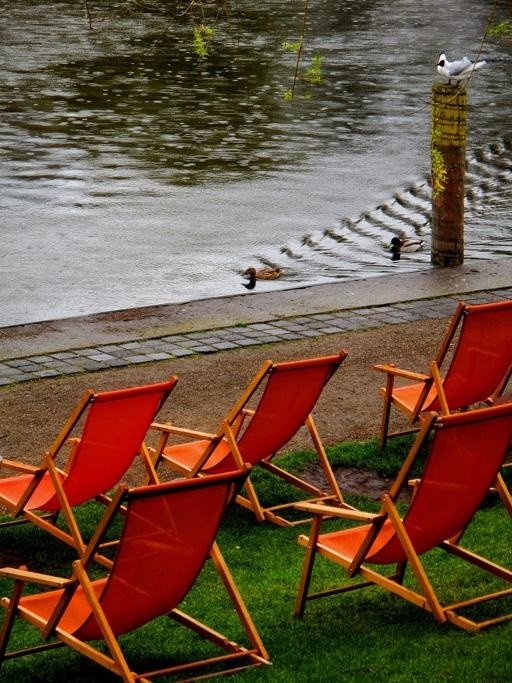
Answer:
[382,237,427,254]
[238,266,285,280]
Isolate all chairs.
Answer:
[0,374,179,576]
[146,348,358,529]
[293,403,512,630]
[373,303,512,450]
[1,461,272,682]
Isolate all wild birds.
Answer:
[433,52,490,90]
